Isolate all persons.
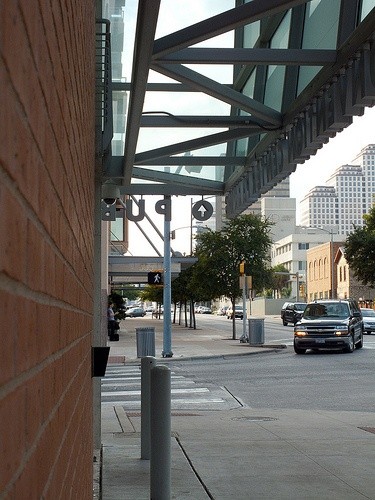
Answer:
[337,306,344,313]
[316,307,323,316]
[108,302,119,336]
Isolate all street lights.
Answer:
[302,226,335,299]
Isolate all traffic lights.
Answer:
[148,271,161,285]
[170,230,175,240]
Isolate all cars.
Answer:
[360,309,375,334]
[124,308,146,317]
[193,306,212,315]
[227,305,247,319]
[216,306,230,316]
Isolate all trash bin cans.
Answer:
[134,326,155,357]
[247,317,264,344]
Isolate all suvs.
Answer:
[280,302,307,327]
[293,298,365,354]
